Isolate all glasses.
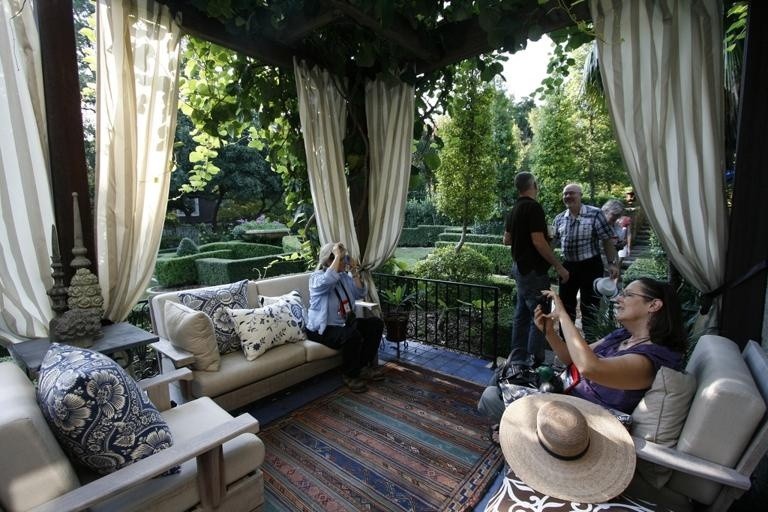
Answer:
[619,288,655,299]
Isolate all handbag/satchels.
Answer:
[496,347,564,408]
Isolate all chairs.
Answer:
[592,330,766,512]
[1,330,269,511]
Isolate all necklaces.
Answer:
[623,337,649,344]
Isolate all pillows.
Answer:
[225,299,304,359]
[34,340,185,479]
[161,299,221,373]
[256,288,309,330]
[174,278,253,357]
[631,367,697,449]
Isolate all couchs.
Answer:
[146,265,383,417]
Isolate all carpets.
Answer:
[252,355,508,512]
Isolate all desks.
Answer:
[482,465,682,512]
[11,319,162,393]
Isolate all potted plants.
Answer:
[378,283,426,344]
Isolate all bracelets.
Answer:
[352,274,358,278]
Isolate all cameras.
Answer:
[615,239,626,250]
[534,292,551,314]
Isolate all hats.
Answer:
[593,278,618,297]
[499,392,637,503]
[319,242,345,264]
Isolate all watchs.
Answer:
[609,261,615,263]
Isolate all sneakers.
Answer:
[341,365,384,392]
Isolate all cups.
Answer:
[547,225,557,239]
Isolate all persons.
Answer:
[597,198,627,326]
[304,242,385,393]
[476,277,687,454]
[551,183,620,346]
[503,171,570,363]
[614,216,632,269]
